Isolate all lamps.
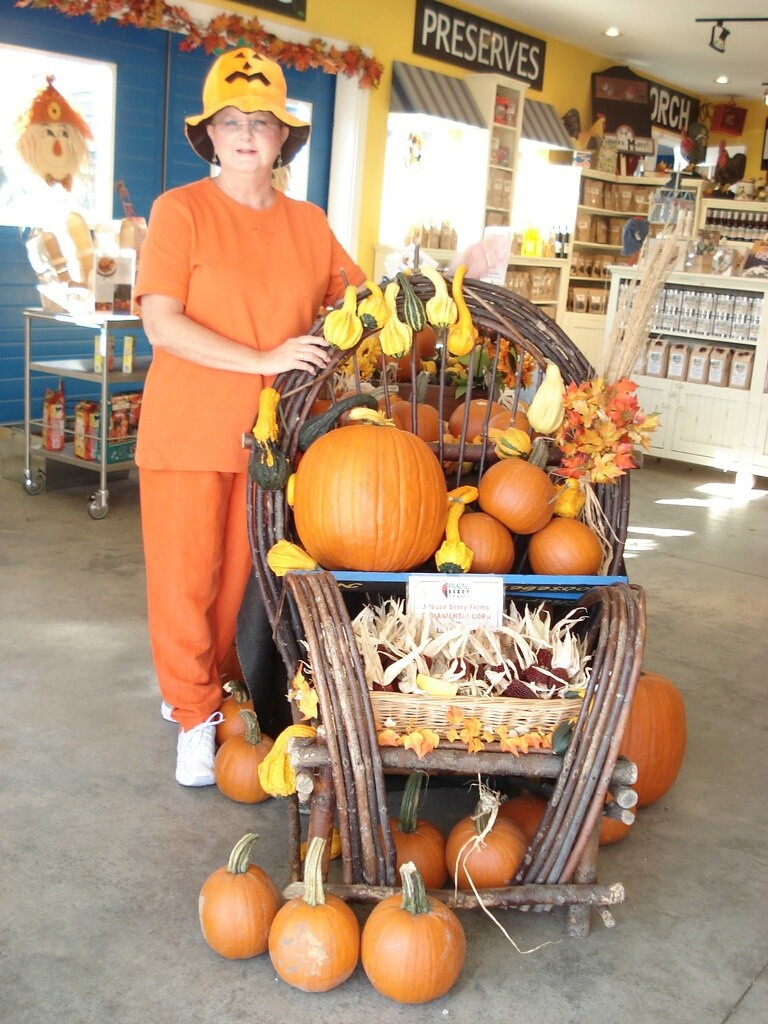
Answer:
[706,23,733,53]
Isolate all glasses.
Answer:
[207,117,282,134]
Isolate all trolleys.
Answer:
[22,306,153,520]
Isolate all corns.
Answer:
[372,642,568,701]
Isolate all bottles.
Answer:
[55,201,95,282]
[555,225,570,258]
[24,226,73,309]
[706,210,768,242]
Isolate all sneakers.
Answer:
[161,700,178,722]
[175,711,226,787]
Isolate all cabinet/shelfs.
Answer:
[22,309,151,519]
[373,74,768,489]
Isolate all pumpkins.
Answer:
[253,263,604,575]
[198,672,685,1004]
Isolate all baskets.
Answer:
[311,605,584,754]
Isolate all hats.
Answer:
[621,215,649,258]
[184,48,312,168]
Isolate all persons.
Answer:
[130,46,380,787]
